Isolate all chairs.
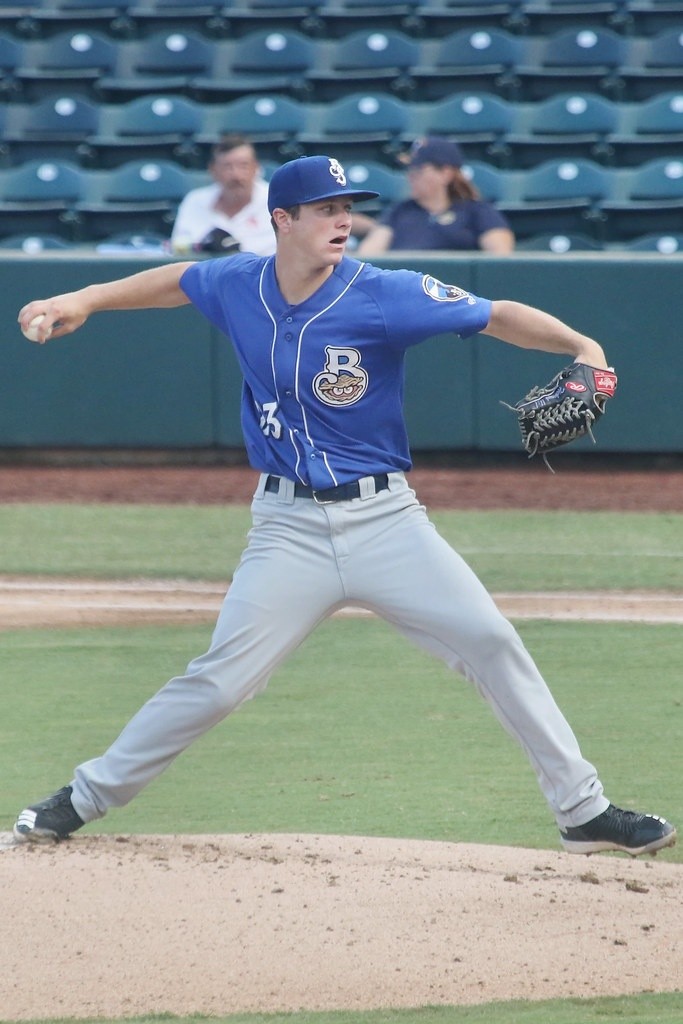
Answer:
[0,0,683,252]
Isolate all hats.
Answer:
[395,138,463,171]
[268,154,380,215]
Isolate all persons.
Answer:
[169,140,276,257]
[356,142,516,258]
[13,155,677,856]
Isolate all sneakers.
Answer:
[557,803,676,858]
[12,784,86,842]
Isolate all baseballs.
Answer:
[21,314,53,342]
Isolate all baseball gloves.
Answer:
[498,362,617,475]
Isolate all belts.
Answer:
[264,472,388,504]
